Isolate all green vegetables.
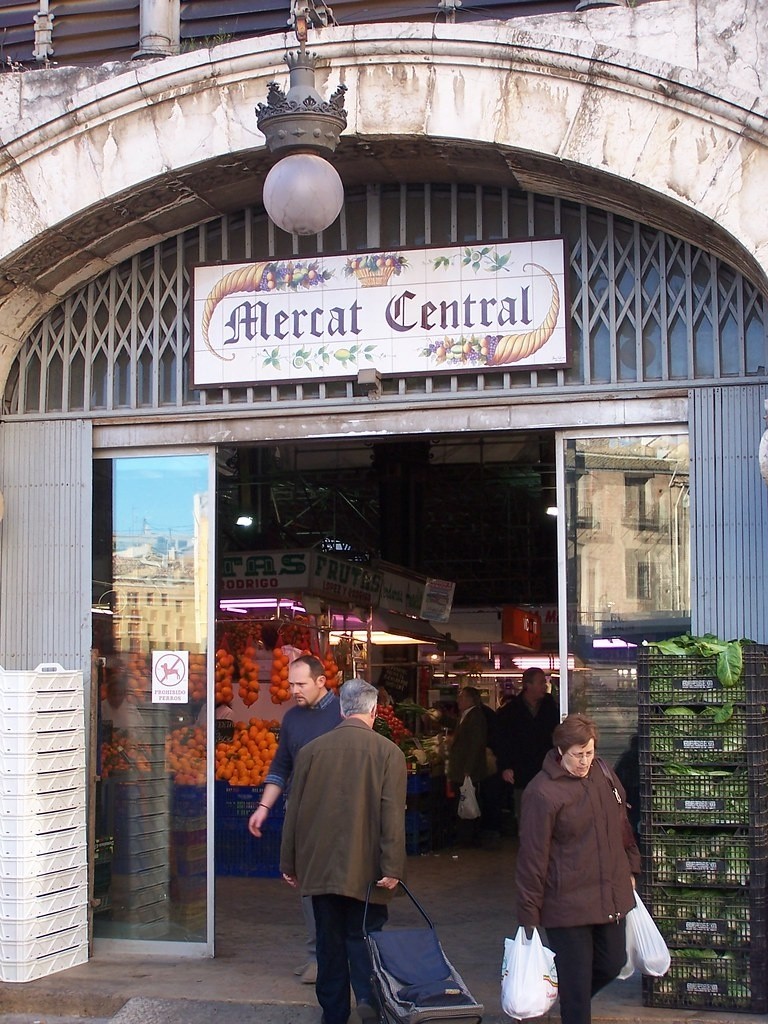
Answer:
[373,716,436,762]
[638,633,765,1006]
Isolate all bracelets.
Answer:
[257,803,272,811]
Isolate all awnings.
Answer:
[329,606,447,646]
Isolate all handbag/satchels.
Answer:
[457,776,481,819]
[615,912,635,980]
[501,925,559,1020]
[595,756,636,847]
[633,888,671,977]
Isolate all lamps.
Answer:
[255,0,349,235]
[437,633,457,654]
[357,368,381,391]
[352,602,370,623]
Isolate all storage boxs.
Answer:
[175,782,287,878]
[633,640,768,1015]
[102,669,206,940]
[403,775,460,853]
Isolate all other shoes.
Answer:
[294,953,310,975]
[301,962,318,982]
[355,996,381,1024]
[321,1009,351,1024]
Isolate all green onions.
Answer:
[392,699,437,719]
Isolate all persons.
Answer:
[247,655,345,984]
[515,713,641,1024]
[279,678,407,1024]
[101,680,146,738]
[420,667,557,857]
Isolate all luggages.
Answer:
[362,879,485,1024]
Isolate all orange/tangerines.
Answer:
[101,613,340,787]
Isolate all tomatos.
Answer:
[377,703,412,744]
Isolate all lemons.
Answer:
[351,259,393,270]
[293,356,305,368]
[266,267,317,289]
[471,252,482,262]
[334,349,351,361]
[436,338,489,362]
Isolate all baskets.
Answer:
[407,772,433,793]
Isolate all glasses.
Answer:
[458,689,468,698]
